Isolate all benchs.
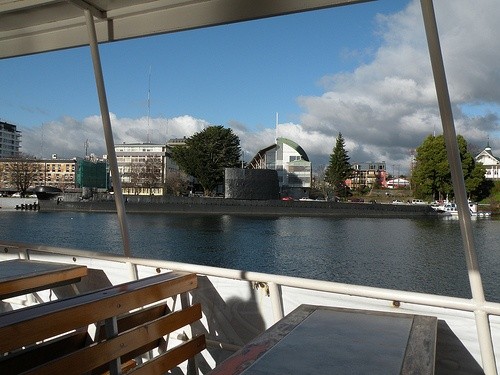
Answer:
[0,271,207,375]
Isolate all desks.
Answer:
[0,258,88,298]
[211,303,439,375]
